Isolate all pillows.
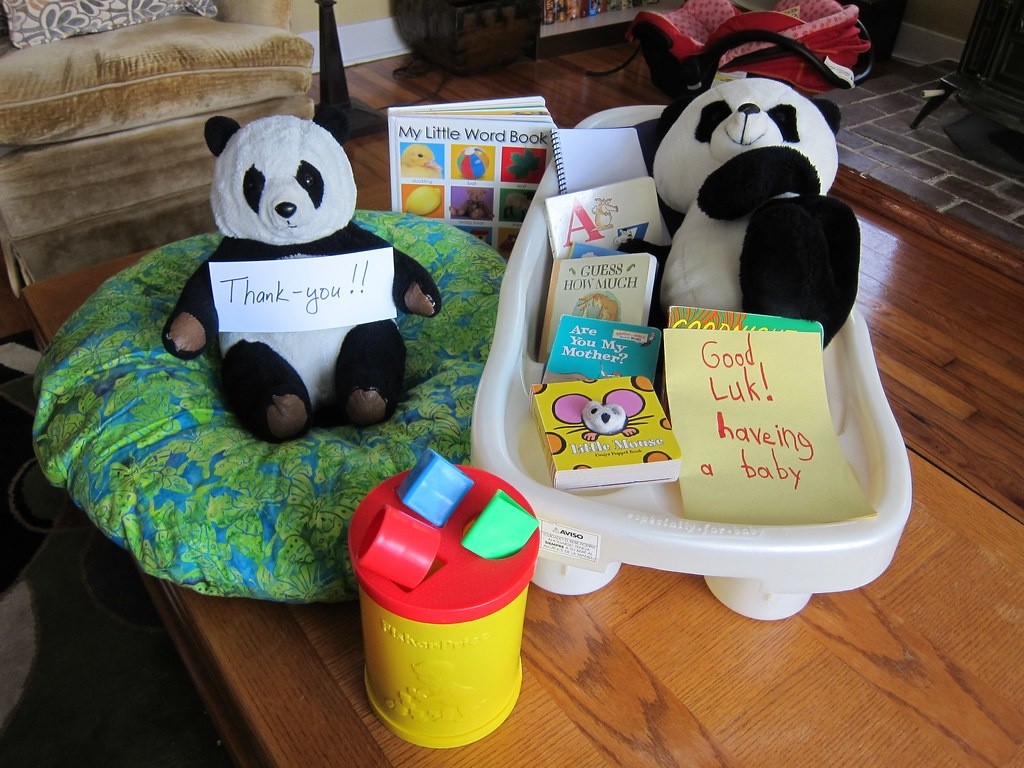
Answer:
[1,0,218,50]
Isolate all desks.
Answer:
[18,164,1024,767]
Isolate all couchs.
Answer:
[0,0,315,285]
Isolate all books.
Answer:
[545,175,672,262]
[529,375,682,491]
[544,0,660,24]
[550,128,672,246]
[660,306,824,422]
[542,314,662,387]
[536,242,658,362]
[387,95,558,262]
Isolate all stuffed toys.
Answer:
[160,106,443,442]
[616,76,860,364]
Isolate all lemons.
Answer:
[406,186,442,216]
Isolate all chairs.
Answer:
[625,0,875,95]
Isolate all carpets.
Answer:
[942,112,1024,173]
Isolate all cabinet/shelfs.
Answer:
[525,1,685,62]
